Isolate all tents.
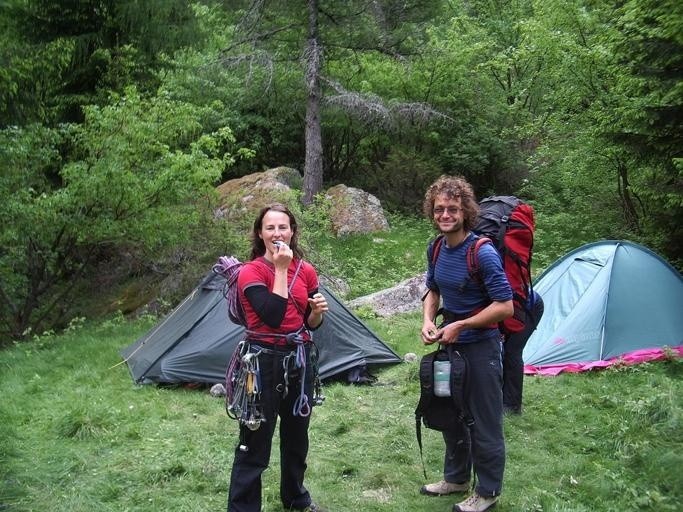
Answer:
[525,241,683,376]
[120,256,406,389]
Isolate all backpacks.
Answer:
[433,196,533,331]
[415,350,475,432]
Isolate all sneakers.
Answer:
[420,481,470,495]
[453,492,499,512]
[299,503,326,512]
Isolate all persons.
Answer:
[226,204,329,511]
[421,174,515,512]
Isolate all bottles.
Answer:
[431,349,452,396]
